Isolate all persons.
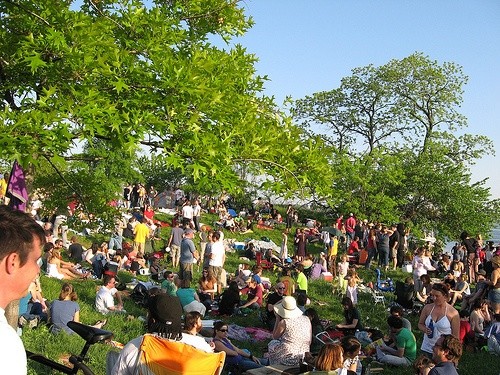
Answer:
[0,171,500,375]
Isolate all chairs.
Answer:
[368,268,417,315]
[130,333,226,375]
[254,250,275,273]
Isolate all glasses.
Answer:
[435,343,442,347]
[203,271,208,273]
[279,287,285,289]
[433,283,448,293]
[170,276,173,278]
[218,329,228,332]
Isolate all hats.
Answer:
[349,213,353,216]
[274,296,303,318]
[150,293,182,321]
[492,256,500,268]
[368,223,375,226]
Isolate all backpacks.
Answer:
[93,256,103,279]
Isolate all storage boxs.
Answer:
[322,272,333,282]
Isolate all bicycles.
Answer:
[24,321,114,375]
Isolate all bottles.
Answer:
[427,319,434,338]
